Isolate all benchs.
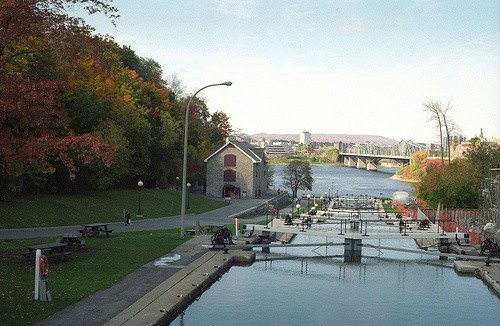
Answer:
[78,228,112,233]
[24,239,72,259]
[207,224,223,232]
[80,239,85,245]
[185,230,196,234]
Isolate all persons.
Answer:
[273,207,279,217]
[125,210,131,226]
[277,189,280,195]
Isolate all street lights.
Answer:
[296,204,301,220]
[307,194,311,215]
[328,188,331,198]
[176,177,180,191]
[179,81,233,238]
[320,196,323,211]
[136,181,143,218]
[186,182,192,212]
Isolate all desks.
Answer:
[28,242,68,265]
[63,233,82,249]
[83,223,109,236]
